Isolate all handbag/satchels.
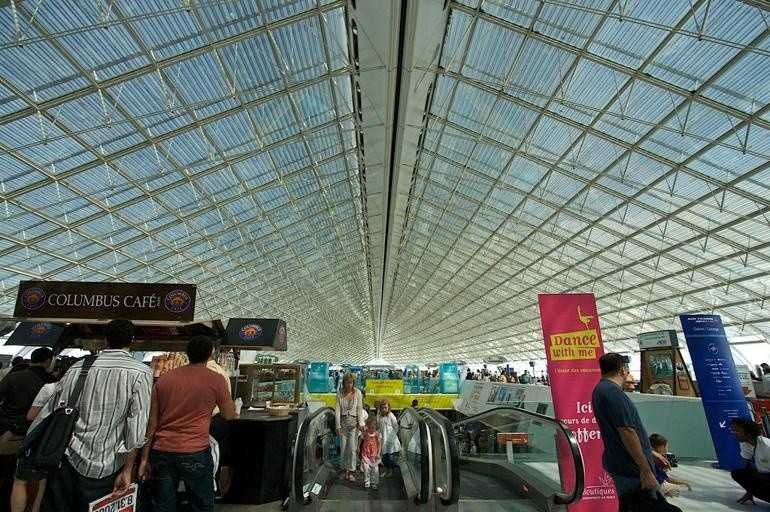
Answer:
[15,407,79,481]
[0,429,26,456]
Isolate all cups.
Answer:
[150,351,177,377]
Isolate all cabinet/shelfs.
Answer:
[199,406,309,507]
[241,358,303,405]
